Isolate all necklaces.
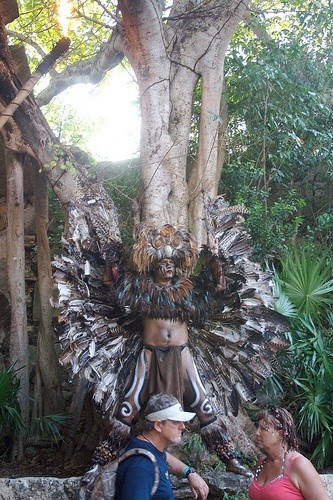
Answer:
[141,433,152,443]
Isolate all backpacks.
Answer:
[79,449,160,500]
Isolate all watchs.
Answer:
[186,467,197,477]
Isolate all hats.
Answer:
[145,403,196,421]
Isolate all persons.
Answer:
[101,393,210,500]
[79,238,256,483]
[249,407,330,500]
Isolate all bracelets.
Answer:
[181,465,190,477]
